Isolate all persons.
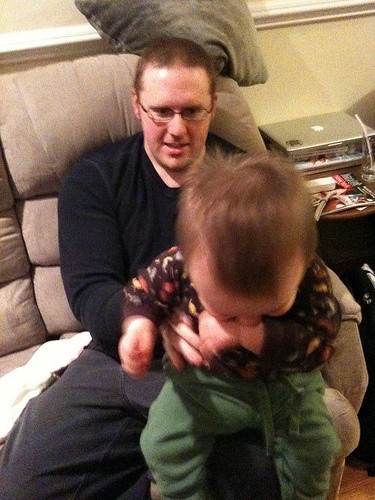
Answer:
[0,38,281,500]
[117,153,342,500]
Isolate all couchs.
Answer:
[0,53,370,500]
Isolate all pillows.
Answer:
[74,0,269,88]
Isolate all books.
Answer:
[305,172,375,217]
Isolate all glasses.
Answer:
[135,94,215,122]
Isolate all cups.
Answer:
[362,136,375,182]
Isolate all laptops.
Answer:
[258,110,375,163]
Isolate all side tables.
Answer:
[299,162,375,266]
